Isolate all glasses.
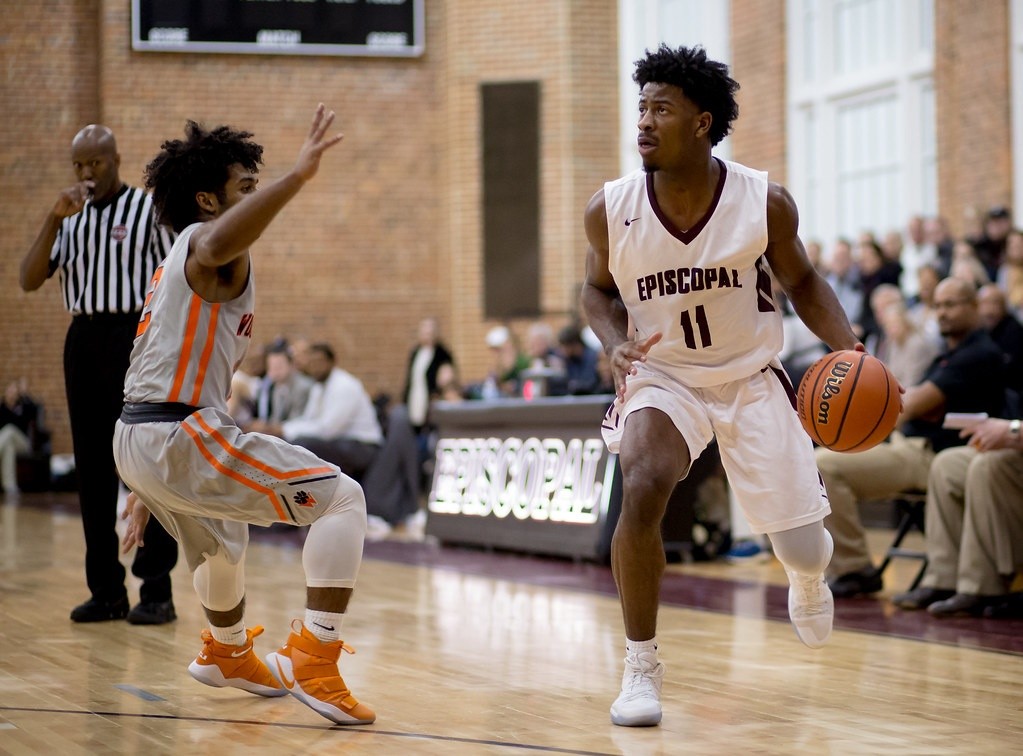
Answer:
[931,299,964,309]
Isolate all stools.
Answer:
[858,489,937,592]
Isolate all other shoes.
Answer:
[70,591,131,624]
[927,593,1011,617]
[830,569,883,597]
[892,585,956,610]
[127,600,179,626]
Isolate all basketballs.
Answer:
[795,348,901,453]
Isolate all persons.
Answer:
[17,123,183,627]
[576,39,910,731]
[227,337,385,472]
[0,379,49,503]
[653,206,1023,620]
[107,99,371,726]
[400,305,615,436]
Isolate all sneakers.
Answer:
[787,570,834,650]
[266,619,376,724]
[609,652,666,726]
[187,624,292,698]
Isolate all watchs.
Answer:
[1009,419,1021,445]
